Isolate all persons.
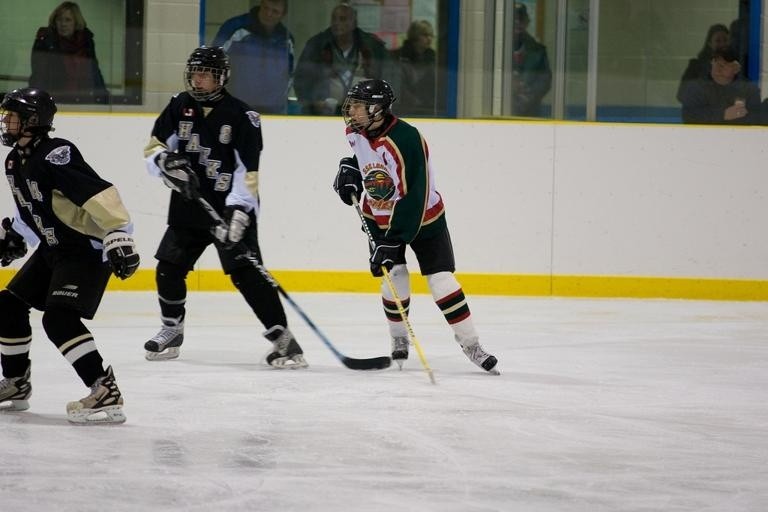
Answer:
[512,3,552,112]
[685,22,730,78]
[759,99,768,127]
[386,21,436,116]
[676,48,759,124]
[143,46,306,364]
[334,79,498,372]
[30,1,107,93]
[0,86,140,416]
[297,2,397,111]
[729,19,742,43]
[208,1,295,115]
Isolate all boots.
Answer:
[144,309,186,352]
[0,361,33,402]
[262,323,302,366]
[65,364,125,418]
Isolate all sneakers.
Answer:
[392,335,410,358]
[455,335,497,371]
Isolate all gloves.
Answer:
[370,238,400,277]
[334,158,364,207]
[159,150,200,199]
[0,217,30,267]
[104,231,140,280]
[210,207,251,253]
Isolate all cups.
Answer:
[735,96,745,106]
[325,97,338,115]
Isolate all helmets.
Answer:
[0,89,56,147]
[183,45,232,102]
[342,79,393,132]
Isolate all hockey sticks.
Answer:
[352,195,436,382]
[194,193,392,370]
